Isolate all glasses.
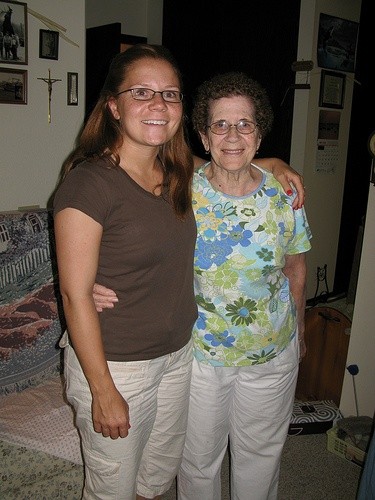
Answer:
[204,120,263,135]
[113,88,184,103]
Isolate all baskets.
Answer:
[326,426,367,468]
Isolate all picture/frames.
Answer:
[38,29,59,60]
[0,67,28,105]
[0,0,28,66]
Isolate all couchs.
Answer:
[0,207,87,500]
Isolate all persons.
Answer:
[92,72,313,500]
[0,31,21,61]
[53,43,308,500]
